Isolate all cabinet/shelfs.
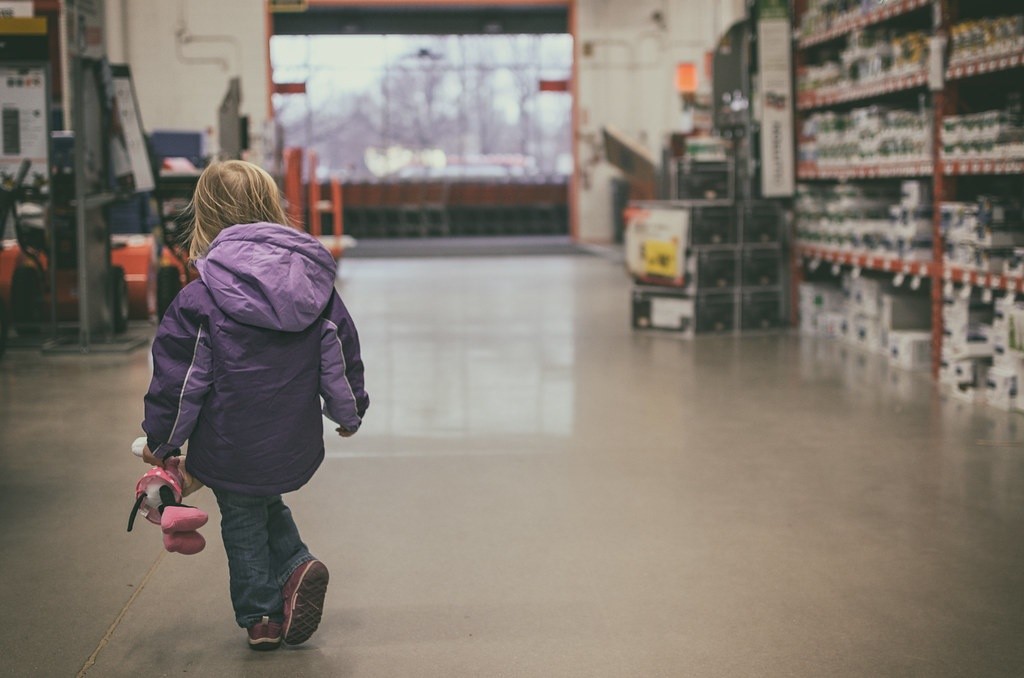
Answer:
[790,0,1024,382]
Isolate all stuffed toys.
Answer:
[127,437,210,556]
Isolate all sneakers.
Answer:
[247,616,281,650]
[280,561,330,645]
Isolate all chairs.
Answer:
[150,131,201,159]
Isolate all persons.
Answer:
[142,158,372,646]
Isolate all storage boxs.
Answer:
[796,18,1024,414]
[624,156,795,340]
